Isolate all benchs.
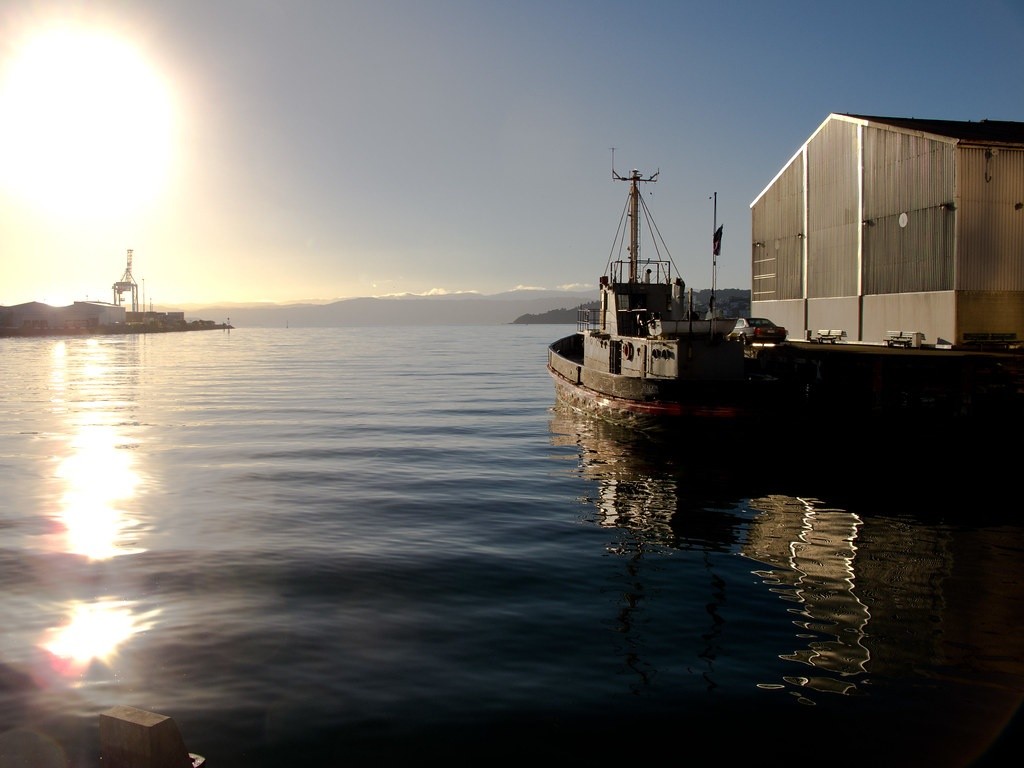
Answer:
[962,333,1024,352]
[883,331,917,348]
[810,330,843,344]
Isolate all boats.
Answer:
[546,147,755,434]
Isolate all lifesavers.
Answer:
[622,342,634,360]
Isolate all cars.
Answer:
[728,318,786,344]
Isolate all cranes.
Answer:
[113,248,141,314]
